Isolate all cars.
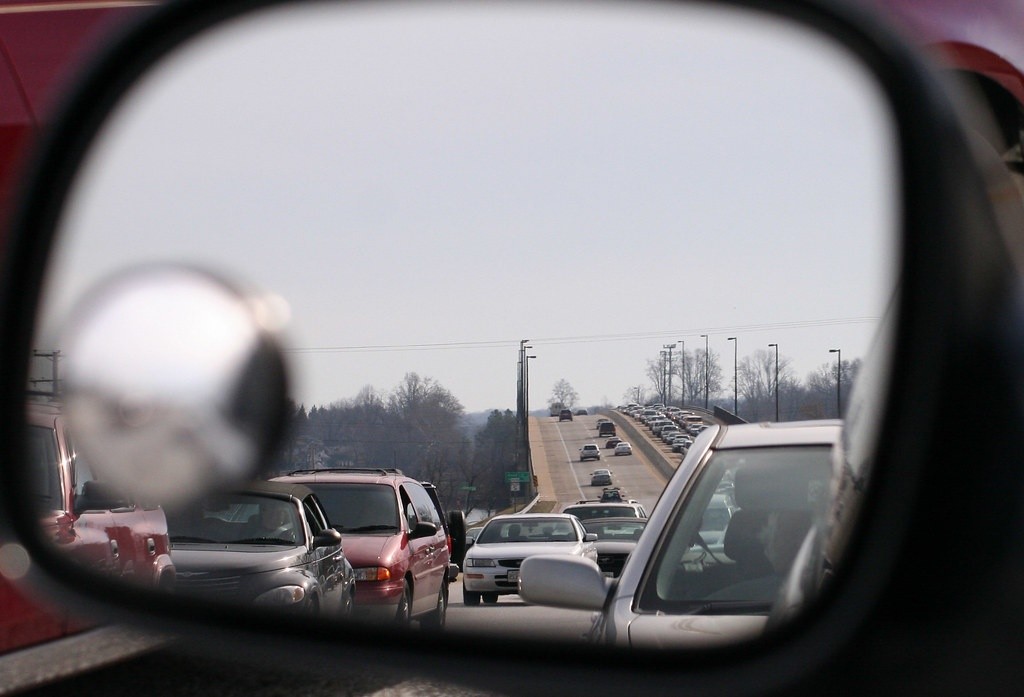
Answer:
[24,404,179,592]
[550,395,741,577]
[515,417,852,647]
[457,511,599,609]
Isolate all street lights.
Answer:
[701,334,710,410]
[829,348,842,418]
[728,337,738,417]
[513,338,536,438]
[678,340,686,409]
[768,342,780,423]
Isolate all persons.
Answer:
[236,506,294,541]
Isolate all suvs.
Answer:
[170,479,358,623]
[223,463,457,641]
